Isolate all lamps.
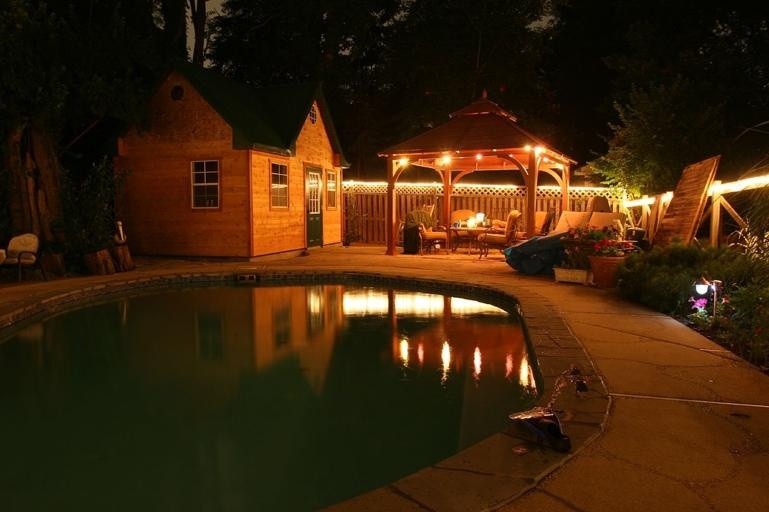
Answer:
[694,272,715,296]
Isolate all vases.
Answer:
[552,267,593,286]
[587,256,624,289]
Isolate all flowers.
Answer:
[559,224,626,271]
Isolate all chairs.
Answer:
[535,209,628,246]
[0,233,47,282]
[404,210,525,260]
